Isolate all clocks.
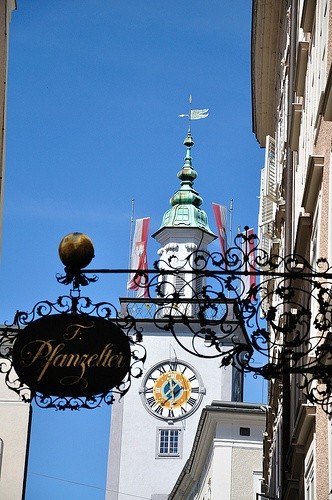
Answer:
[141,357,209,424]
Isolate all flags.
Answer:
[240,227,257,302]
[127,217,151,291]
[135,242,151,298]
[212,203,230,270]
[190,109,209,121]
